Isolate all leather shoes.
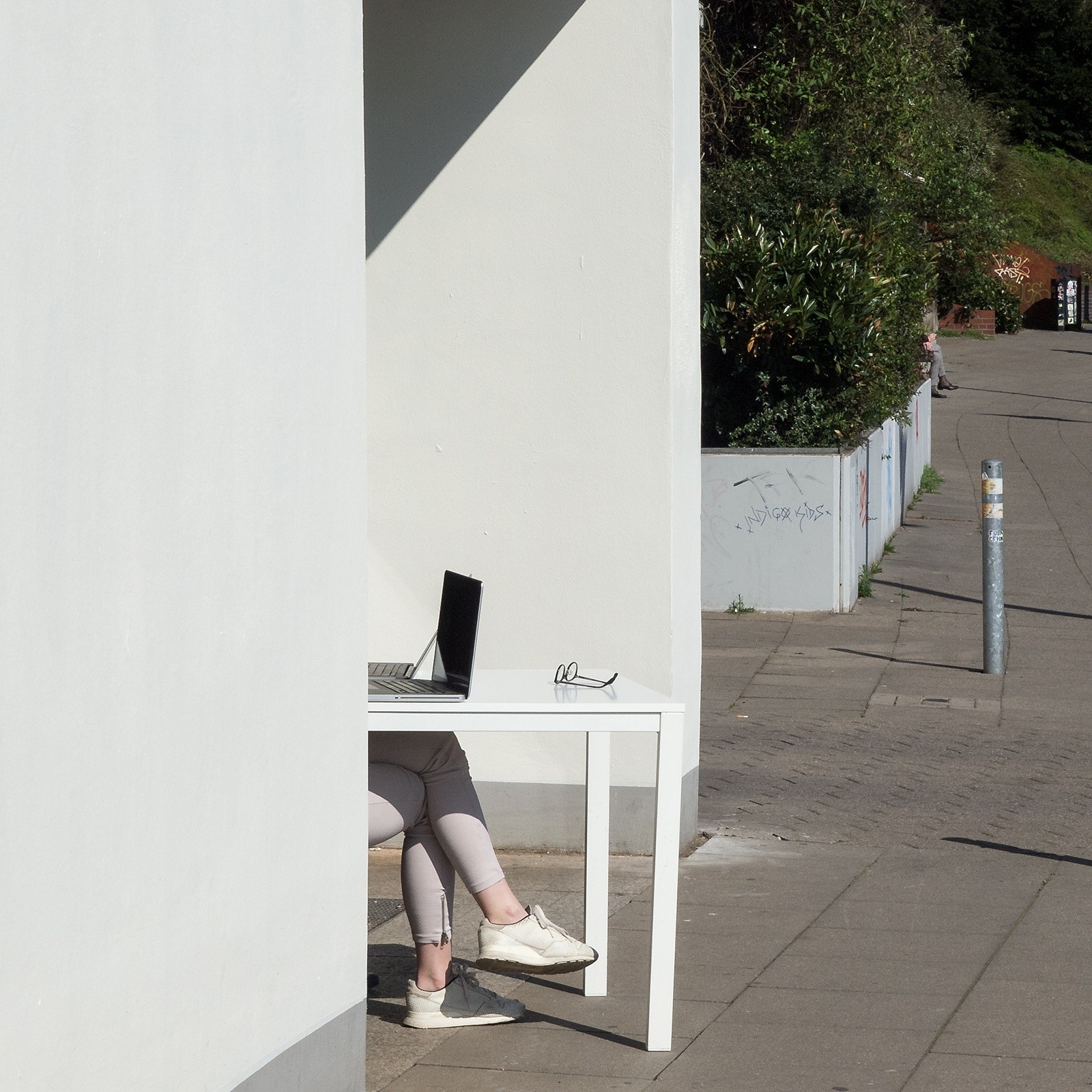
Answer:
[938,383,958,390]
[932,392,947,398]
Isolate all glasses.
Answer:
[553,663,618,688]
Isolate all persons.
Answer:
[368,731,599,1028]
[915,333,959,398]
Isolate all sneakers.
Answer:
[403,964,524,1029]
[476,907,598,976]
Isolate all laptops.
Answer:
[368,570,483,702]
